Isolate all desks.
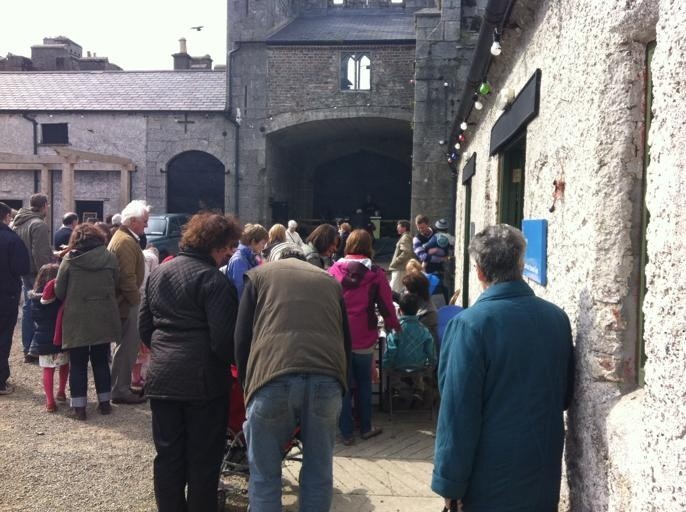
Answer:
[367,301,403,410]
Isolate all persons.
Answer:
[1,191,159,420]
[234,239,348,512]
[430,224,577,512]
[222,220,306,292]
[136,208,239,512]
[307,208,453,448]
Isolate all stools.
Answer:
[391,357,440,421]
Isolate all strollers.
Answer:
[216,365,304,511]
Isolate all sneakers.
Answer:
[0,387,13,395]
[64,407,85,420]
[46,405,56,412]
[95,401,111,414]
[55,397,65,405]
[112,393,145,404]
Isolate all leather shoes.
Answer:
[362,421,384,438]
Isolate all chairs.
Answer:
[447,289,461,307]
[433,304,464,359]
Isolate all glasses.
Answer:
[46,202,51,209]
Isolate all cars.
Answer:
[146,214,189,263]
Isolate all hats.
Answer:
[435,218,448,230]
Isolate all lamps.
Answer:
[446,26,507,168]
